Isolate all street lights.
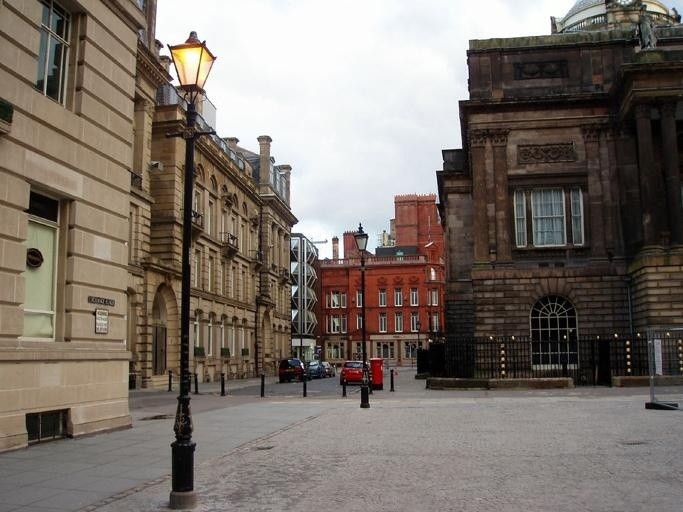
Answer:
[416,320,421,349]
[352,222,369,408]
[165,31,217,509]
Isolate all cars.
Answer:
[321,362,336,377]
[303,359,326,379]
[339,360,371,386]
[278,357,312,382]
